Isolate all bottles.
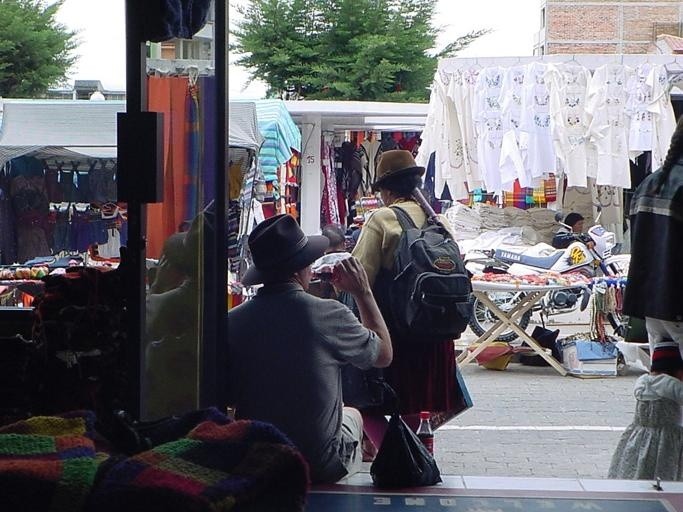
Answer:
[416,411,434,460]
[311,263,341,283]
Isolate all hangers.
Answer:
[384,133,399,150]
[470,53,683,72]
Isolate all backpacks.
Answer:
[388,204,478,343]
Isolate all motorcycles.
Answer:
[467,203,631,344]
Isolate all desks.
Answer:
[457,280,584,376]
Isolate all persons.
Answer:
[607,338,683,481]
[619,114,682,370]
[309,223,358,318]
[140,209,215,430]
[348,147,454,457]
[550,212,597,251]
[222,212,397,482]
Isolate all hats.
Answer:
[235,210,334,286]
[366,148,426,192]
